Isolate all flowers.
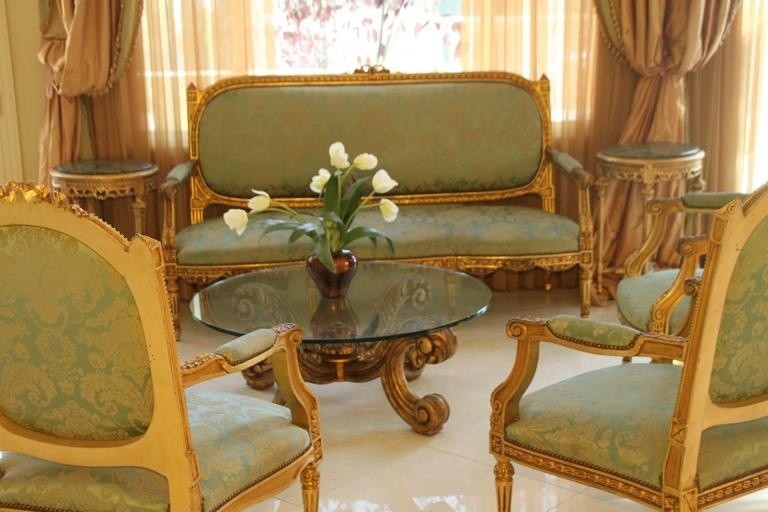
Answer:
[224,142,400,273]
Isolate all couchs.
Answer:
[161,63,595,343]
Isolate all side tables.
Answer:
[594,141,706,308]
[49,159,160,236]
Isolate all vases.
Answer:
[310,297,360,338]
[307,249,358,298]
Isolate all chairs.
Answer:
[616,193,753,363]
[489,183,768,512]
[0,180,323,512]
